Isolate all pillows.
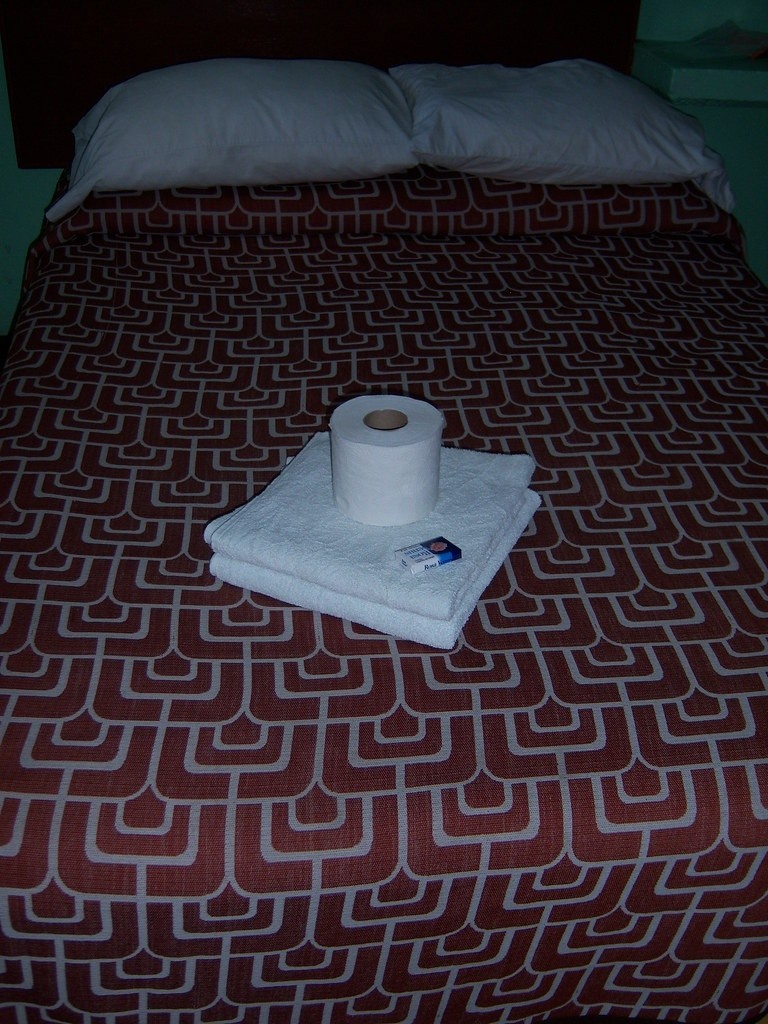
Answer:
[389,59,736,213]
[47,59,420,223]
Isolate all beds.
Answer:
[0,0,768,1024]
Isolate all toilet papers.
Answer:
[327,394,447,528]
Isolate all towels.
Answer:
[204,431,541,649]
